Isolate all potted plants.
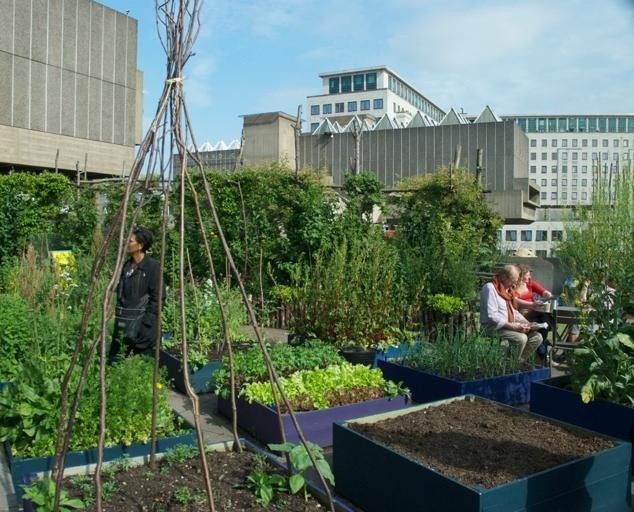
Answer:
[266,219,430,368]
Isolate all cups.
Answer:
[543,303,551,312]
[548,297,557,311]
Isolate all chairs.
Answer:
[468,299,510,347]
[548,308,611,377]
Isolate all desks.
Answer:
[529,302,579,367]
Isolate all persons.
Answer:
[507,264,552,364]
[107,228,166,365]
[568,269,616,343]
[480,264,544,364]
